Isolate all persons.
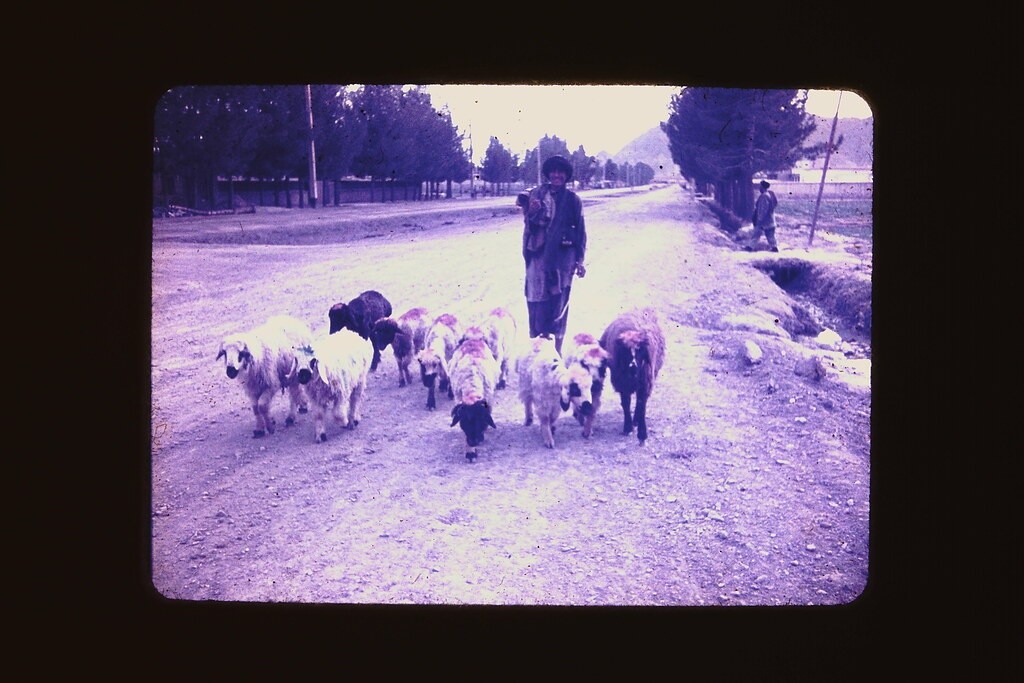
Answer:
[517,153,589,353]
[742,179,778,254]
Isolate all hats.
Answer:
[541,156,573,180]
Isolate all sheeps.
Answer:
[328,290,392,373]
[457,309,516,390]
[447,338,501,463]
[600,307,664,447]
[215,314,317,439]
[372,308,432,388]
[418,313,462,411]
[285,326,375,443]
[564,332,609,440]
[514,331,579,449]
